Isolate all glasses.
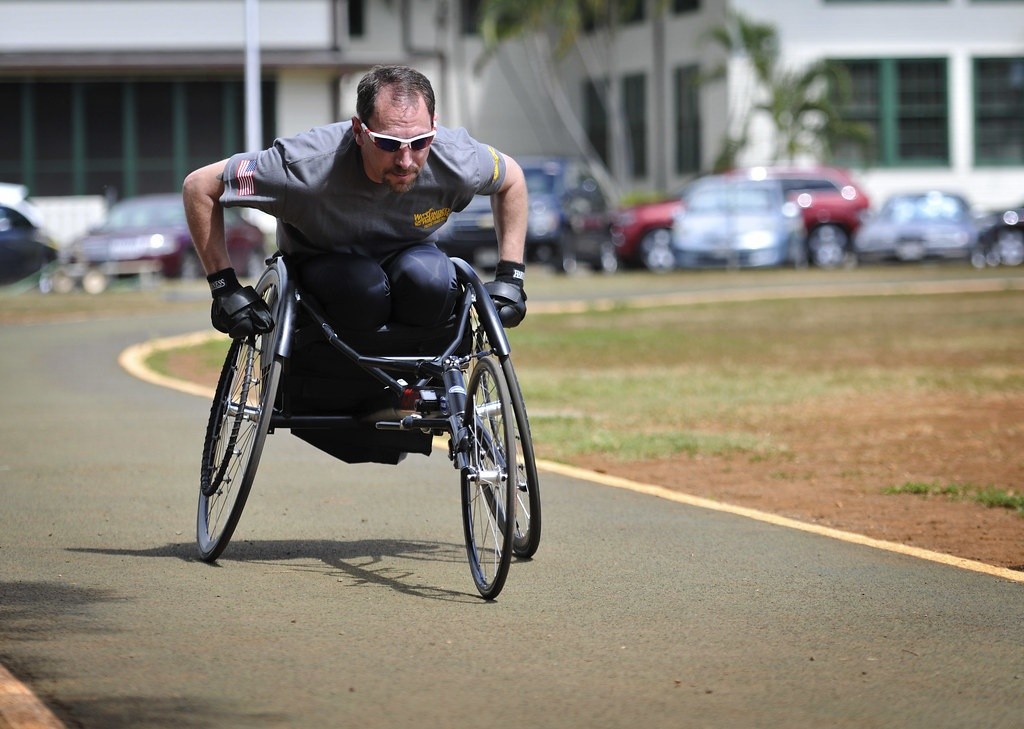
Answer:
[358,116,436,152]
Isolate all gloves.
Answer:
[207,268,275,345]
[482,260,527,328]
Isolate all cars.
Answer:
[0,151,1024,298]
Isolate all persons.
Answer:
[182,66,529,379]
